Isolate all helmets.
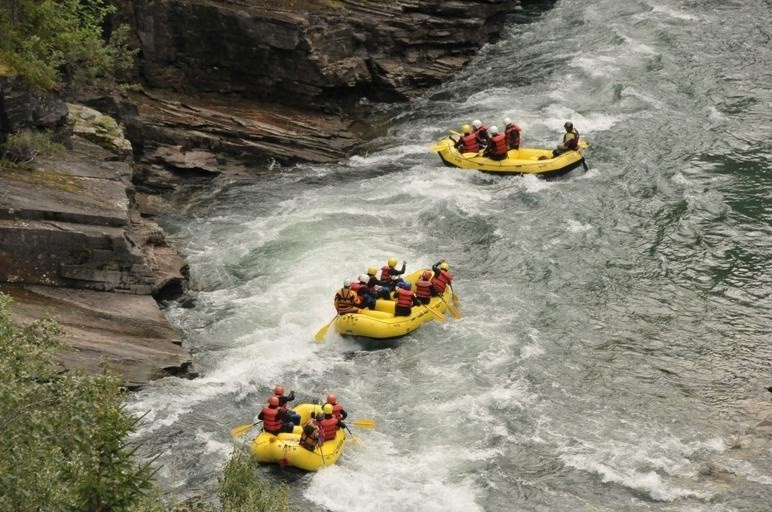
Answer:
[323,403,333,414]
[404,282,411,289]
[344,279,350,287]
[270,397,279,405]
[368,267,377,275]
[440,263,448,271]
[327,394,336,402]
[564,122,573,129]
[317,411,325,420]
[275,386,283,394]
[489,126,498,134]
[472,120,482,130]
[463,125,470,133]
[502,117,511,126]
[422,271,432,280]
[360,274,370,284]
[388,258,397,267]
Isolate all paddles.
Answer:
[449,129,461,136]
[431,144,454,151]
[341,419,376,429]
[231,420,264,436]
[421,302,445,321]
[345,424,362,446]
[314,314,338,342]
[438,294,461,319]
[578,141,590,147]
[450,284,459,308]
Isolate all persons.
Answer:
[334,257,453,316]
[257,387,347,451]
[454,117,522,161]
[552,121,579,156]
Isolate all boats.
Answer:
[334,269,455,341]
[251,401,347,472]
[435,129,592,175]
